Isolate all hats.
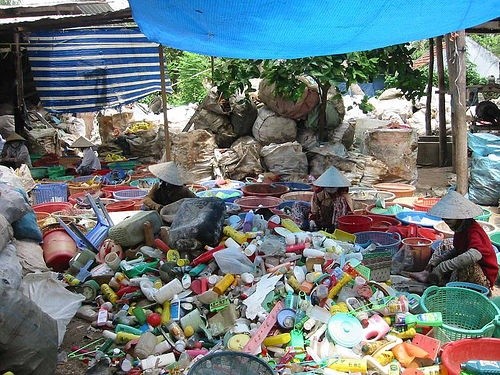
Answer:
[147,160,183,186]
[4,133,28,142]
[69,136,96,148]
[311,165,353,188]
[425,190,484,220]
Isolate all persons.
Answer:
[141,160,200,211]
[0,131,33,171]
[69,136,102,173]
[399,189,499,298]
[309,167,353,230]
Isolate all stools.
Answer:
[55,193,115,249]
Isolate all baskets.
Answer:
[189,177,500,375]
[27,156,159,237]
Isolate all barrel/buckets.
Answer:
[42,227,77,270]
[338,215,373,234]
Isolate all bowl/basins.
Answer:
[348,182,500,251]
[195,179,313,212]
[105,200,136,212]
[30,166,49,178]
[101,187,139,199]
[30,201,73,214]
[441,338,500,375]
[30,154,45,161]
[107,161,141,170]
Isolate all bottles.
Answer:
[62,215,500,375]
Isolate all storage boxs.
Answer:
[32,183,68,204]
